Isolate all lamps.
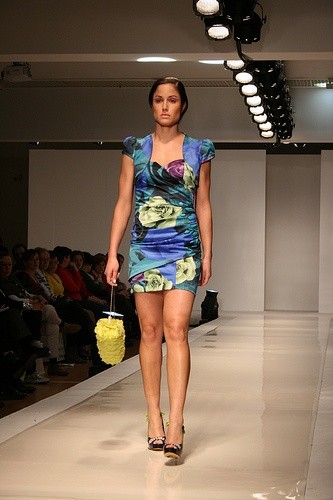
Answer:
[192,0,296,139]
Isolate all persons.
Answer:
[104,77,215,459]
[0,243,140,401]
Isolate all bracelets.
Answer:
[94,297,99,303]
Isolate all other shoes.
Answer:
[48,365,68,376]
[20,368,50,384]
[78,351,89,359]
[18,332,49,357]
[0,389,27,400]
[59,322,82,334]
[59,354,78,363]
[10,376,31,393]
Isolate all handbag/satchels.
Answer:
[94,282,126,366]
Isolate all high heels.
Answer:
[164,426,184,459]
[148,412,166,451]
